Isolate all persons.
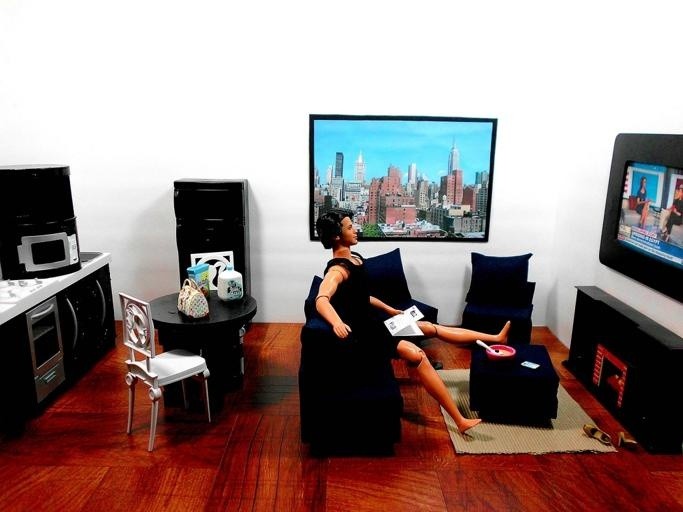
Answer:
[661,188,683,242]
[636,176,651,229]
[316,210,512,432]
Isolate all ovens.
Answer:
[25,295,67,404]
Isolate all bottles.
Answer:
[217,265,244,302]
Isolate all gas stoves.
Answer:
[0,278,59,304]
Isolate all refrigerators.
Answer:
[173,178,252,299]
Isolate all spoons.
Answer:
[476,339,503,355]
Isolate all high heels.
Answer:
[660,229,669,242]
[583,424,611,444]
[617,432,638,448]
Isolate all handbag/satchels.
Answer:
[177,278,209,318]
